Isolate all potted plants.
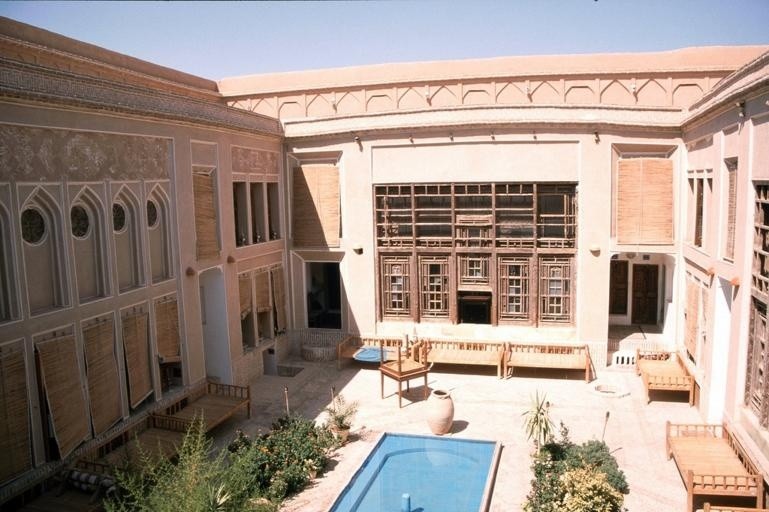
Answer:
[518,389,557,466]
[316,394,359,443]
[303,462,322,479]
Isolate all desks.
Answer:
[378,339,431,408]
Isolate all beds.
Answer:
[634,347,696,407]
[664,420,769,512]
[78,379,251,480]
[336,334,591,382]
